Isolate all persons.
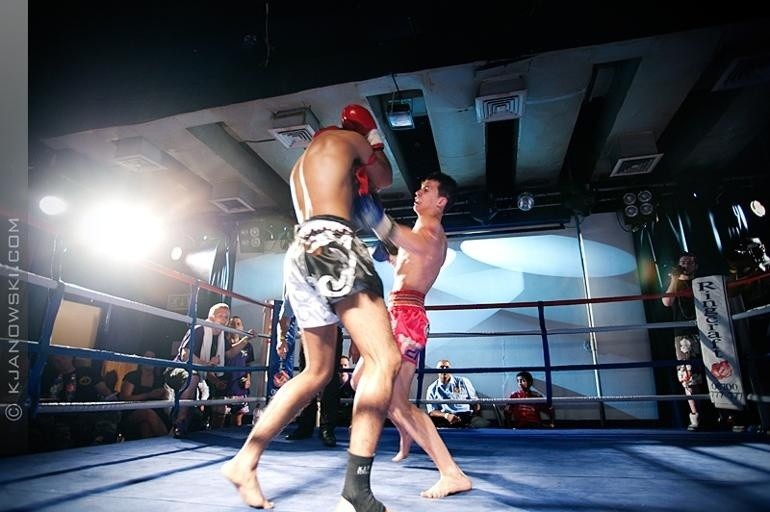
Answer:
[348,168,475,501]
[661,250,717,434]
[219,100,405,512]
[29,282,353,452]
[503,372,555,429]
[423,358,495,429]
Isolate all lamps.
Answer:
[515,190,536,214]
[621,187,655,219]
[382,100,416,129]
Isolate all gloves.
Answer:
[351,193,392,241]
[371,240,393,265]
[340,103,387,151]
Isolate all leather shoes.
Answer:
[318,428,337,447]
[284,427,313,441]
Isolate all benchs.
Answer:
[477,401,510,429]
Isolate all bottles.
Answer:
[49,372,63,400]
[65,371,78,401]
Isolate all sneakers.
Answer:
[687,412,704,432]
[200,413,211,431]
[173,425,186,439]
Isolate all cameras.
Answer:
[672,265,686,277]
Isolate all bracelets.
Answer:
[444,412,449,419]
